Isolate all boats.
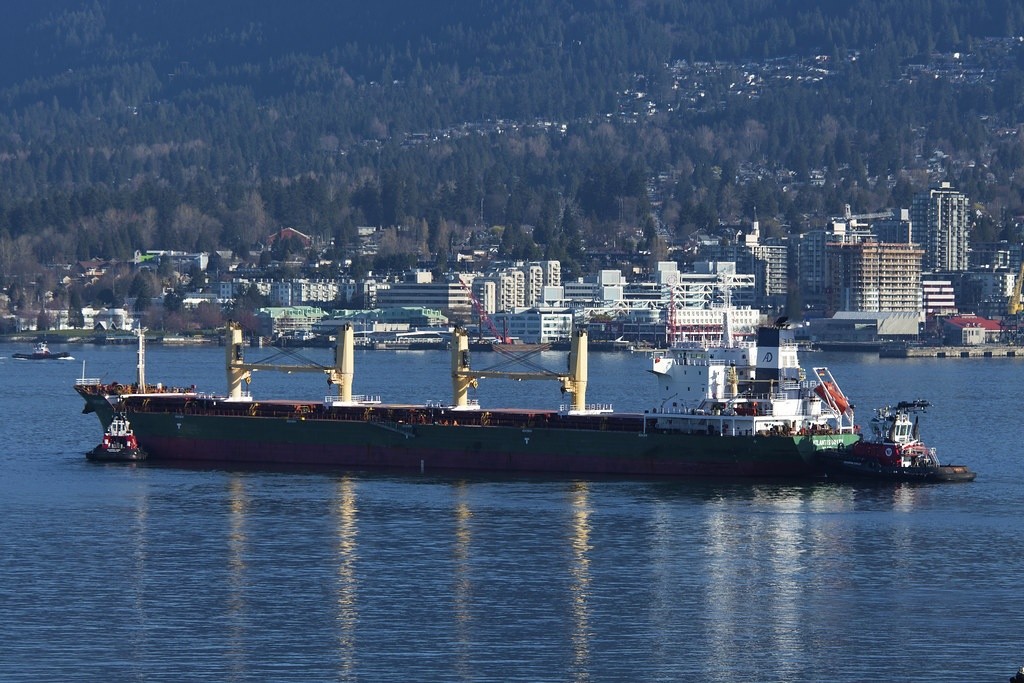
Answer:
[815,396,977,483]
[12,337,70,360]
[85,399,149,461]
[73,312,865,479]
[780,337,823,352]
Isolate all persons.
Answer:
[812,423,816,434]
[131,382,138,394]
[783,423,790,436]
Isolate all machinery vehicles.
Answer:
[457,276,512,345]
